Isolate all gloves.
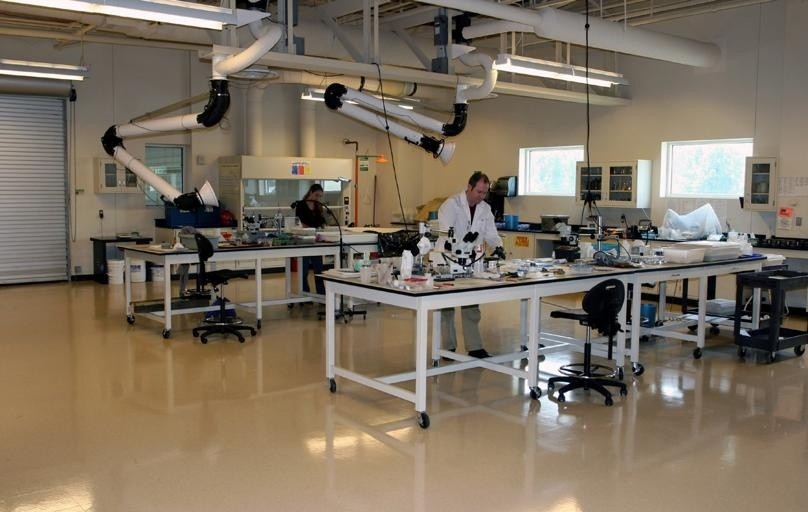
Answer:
[490,247,506,260]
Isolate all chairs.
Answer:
[193,231,257,343]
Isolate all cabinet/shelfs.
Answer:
[575,158,653,210]
[742,157,778,212]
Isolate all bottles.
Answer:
[427,260,450,274]
[473,250,483,273]
[496,265,500,275]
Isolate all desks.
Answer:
[315,222,808,428]
[114,217,439,338]
[90,231,155,285]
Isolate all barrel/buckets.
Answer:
[131,260,146,283]
[642,304,656,328]
[504,215,518,228]
[107,259,124,284]
[151,265,164,281]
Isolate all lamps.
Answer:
[356,155,390,166]
[489,26,630,90]
[0,2,279,82]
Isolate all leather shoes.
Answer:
[305,302,314,305]
[468,349,492,358]
[442,349,456,361]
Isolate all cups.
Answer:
[360,267,371,284]
[489,261,496,269]
[377,262,393,285]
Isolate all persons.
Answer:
[438,171,506,362]
[295,184,327,306]
